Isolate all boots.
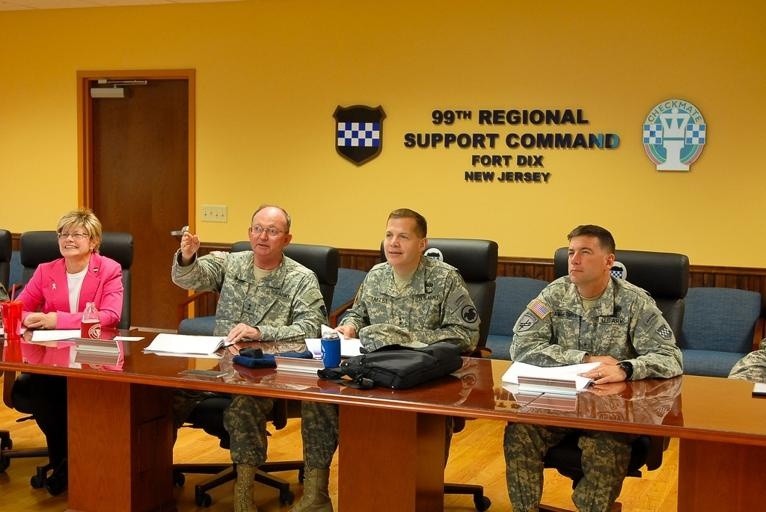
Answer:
[231,463,258,512]
[285,461,335,512]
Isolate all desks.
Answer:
[2,321,766,509]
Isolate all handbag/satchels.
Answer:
[317,341,465,392]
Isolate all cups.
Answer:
[1,302,22,340]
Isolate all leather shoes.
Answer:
[45,468,67,496]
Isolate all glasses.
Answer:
[56,232,89,239]
[250,224,289,236]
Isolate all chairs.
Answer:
[328,262,374,326]
[675,281,764,376]
[331,233,501,511]
[536,245,689,511]
[16,230,136,496]
[8,250,23,292]
[482,271,554,362]
[170,235,341,512]
[0,230,15,468]
[176,285,220,335]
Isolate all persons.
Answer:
[1,204,124,496]
[170,204,327,512]
[502,225,685,512]
[283,208,484,512]
[726,335,766,382]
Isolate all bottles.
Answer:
[80,301,101,338]
